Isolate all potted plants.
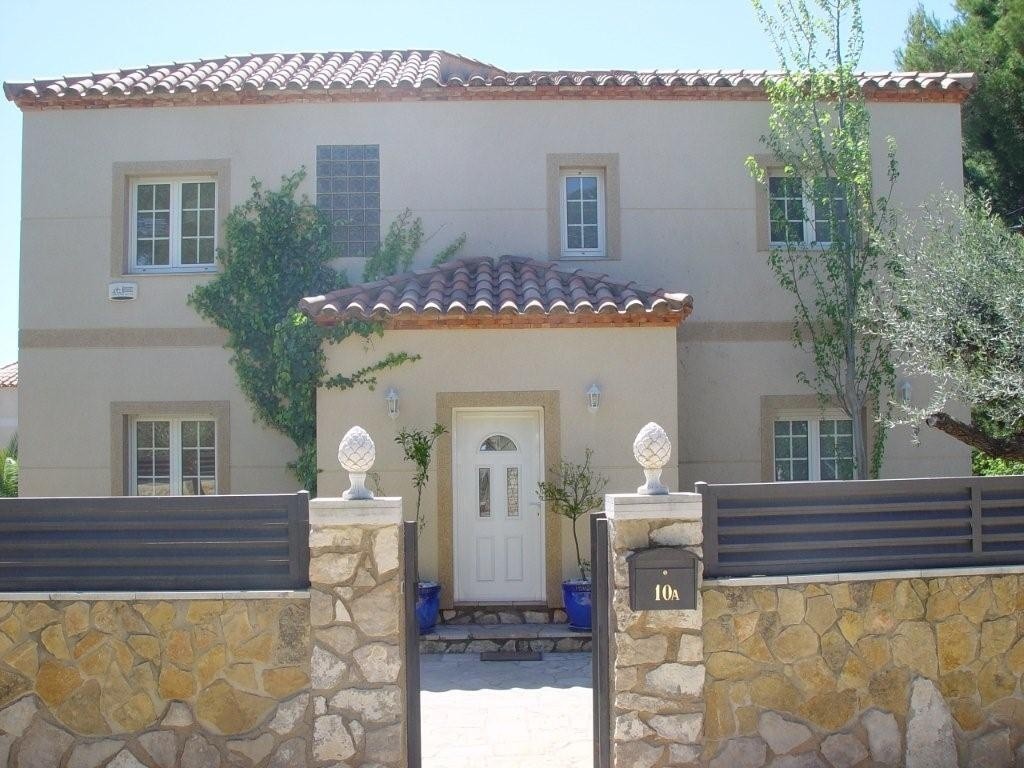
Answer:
[394,422,451,633]
[535,448,610,633]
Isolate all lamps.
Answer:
[385,389,399,421]
[902,382,913,404]
[588,384,602,413]
